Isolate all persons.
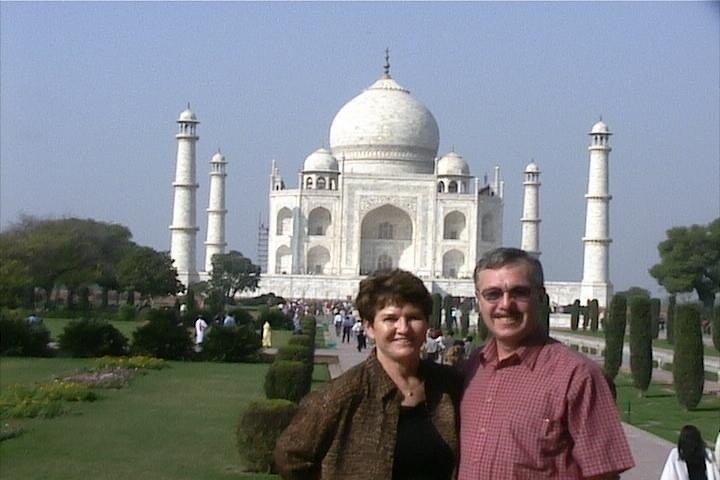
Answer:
[212,313,238,328]
[277,293,478,375]
[659,423,720,480]
[270,267,467,479]
[456,246,637,479]
[194,315,209,346]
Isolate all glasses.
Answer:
[482,287,531,301]
